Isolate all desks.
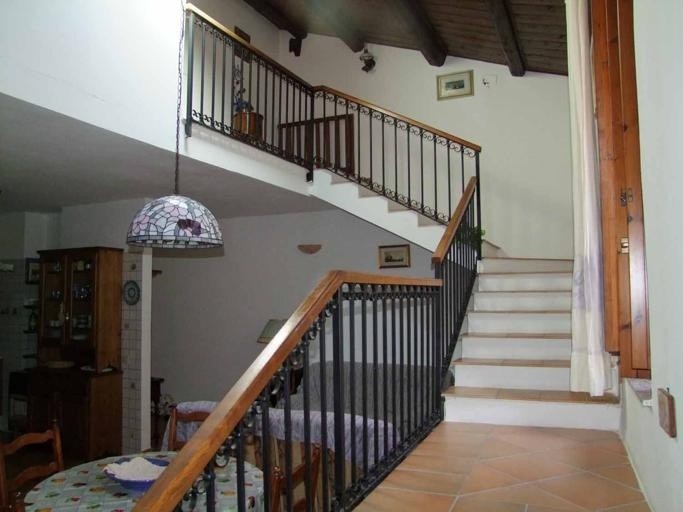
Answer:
[26,448,264,512]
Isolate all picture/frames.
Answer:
[436,69,475,101]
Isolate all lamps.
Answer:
[127,4,222,258]
[359,48,376,72]
[377,244,411,269]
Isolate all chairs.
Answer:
[1,419,65,512]
[170,407,320,511]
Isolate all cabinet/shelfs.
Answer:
[7,247,123,464]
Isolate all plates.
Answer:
[109,455,169,490]
